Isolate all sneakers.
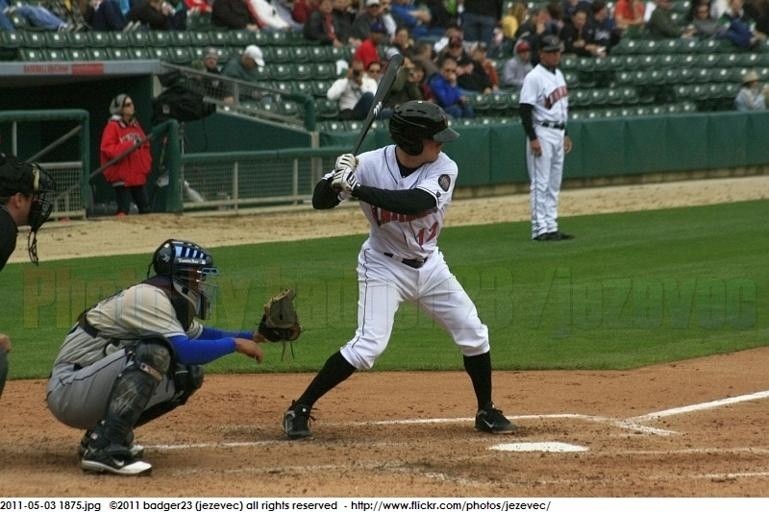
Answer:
[536,233,561,240]
[552,231,573,240]
[79,446,151,477]
[63,22,88,33]
[78,430,144,459]
[474,405,519,435]
[280,398,314,438]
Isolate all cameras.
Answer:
[353,69,361,75]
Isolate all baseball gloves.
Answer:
[258,288,300,344]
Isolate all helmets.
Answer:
[538,33,563,53]
[0,159,58,266]
[152,237,217,320]
[389,100,460,156]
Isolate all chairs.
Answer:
[1,0,768,131]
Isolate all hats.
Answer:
[108,94,127,116]
[516,42,528,54]
[469,42,485,53]
[366,0,379,8]
[369,22,386,31]
[201,46,217,61]
[740,70,759,85]
[244,45,264,66]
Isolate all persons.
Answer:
[0,153,55,400]
[46,239,304,477]
[281,99,518,440]
[519,35,574,241]
[0,1,769,125]
[101,94,154,217]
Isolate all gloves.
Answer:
[332,151,358,172]
[329,168,361,198]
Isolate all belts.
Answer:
[541,122,565,130]
[383,251,428,270]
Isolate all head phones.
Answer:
[114,93,120,107]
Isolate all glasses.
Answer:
[370,70,380,73]
[444,69,455,73]
[448,43,461,49]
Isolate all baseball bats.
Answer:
[332,55,404,193]
